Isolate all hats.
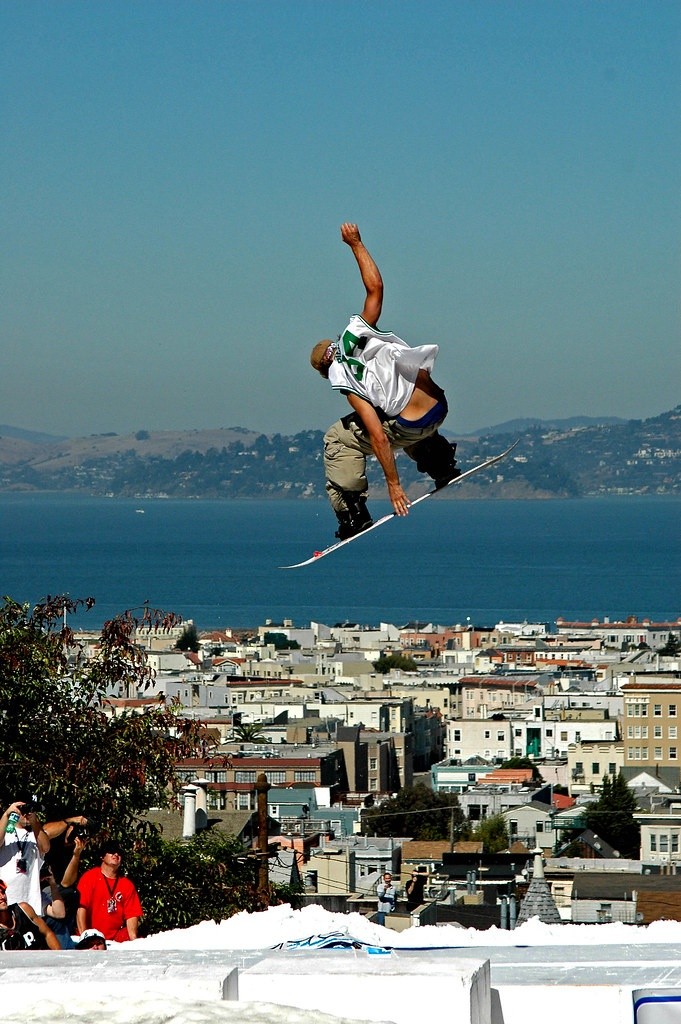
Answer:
[75,928,106,950]
[14,790,42,813]
[99,839,124,855]
[311,340,338,370]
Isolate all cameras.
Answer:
[19,803,37,815]
[388,884,391,887]
[75,820,103,841]
[39,867,51,878]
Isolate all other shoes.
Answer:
[426,463,462,488]
[335,509,373,541]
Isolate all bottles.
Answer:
[6,806,22,833]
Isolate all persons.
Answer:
[377,870,429,926]
[75,841,144,941]
[75,928,107,950]
[311,221,461,540]
[0,790,98,950]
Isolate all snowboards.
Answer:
[278,438,519,570]
